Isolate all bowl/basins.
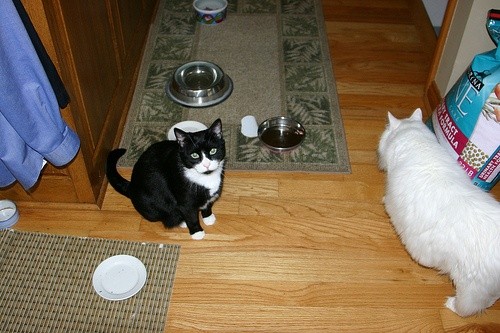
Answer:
[165,60,233,108]
[257,116,306,153]
[0,199,19,231]
[193,0,229,24]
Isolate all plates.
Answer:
[92,255,147,301]
[167,121,208,140]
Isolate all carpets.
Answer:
[116,0,351,173]
[0,231,180,333]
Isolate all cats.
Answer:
[378,108,499,315]
[104,118,226,240]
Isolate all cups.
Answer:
[240,115,258,137]
